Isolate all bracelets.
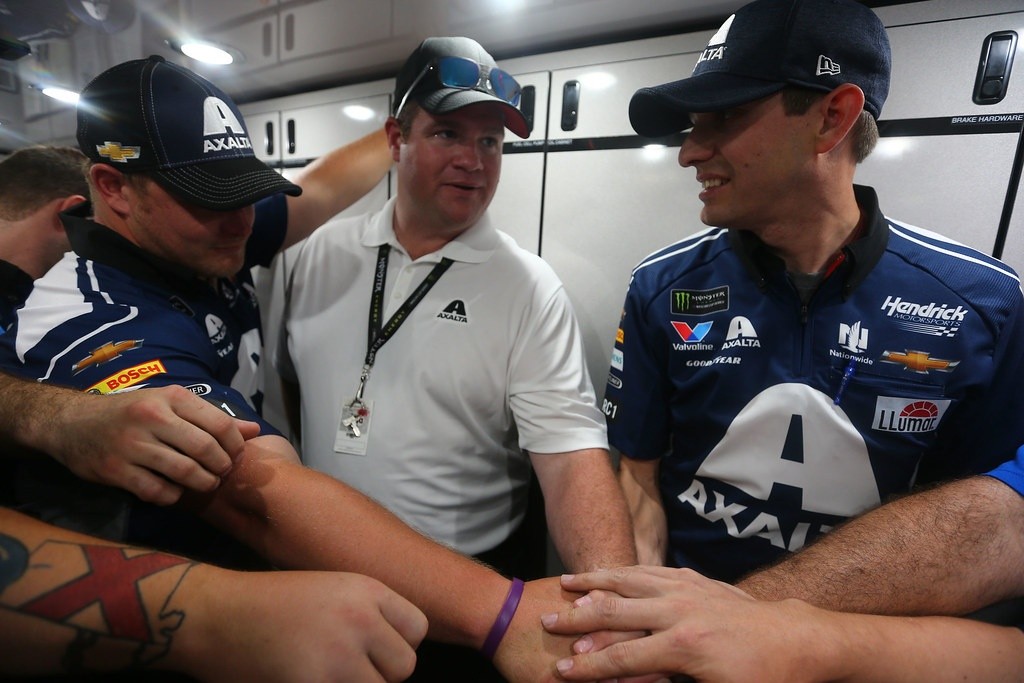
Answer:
[479,576,525,658]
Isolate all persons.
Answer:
[541,0,1024,683]
[0,34,667,683]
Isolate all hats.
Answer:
[629,0,891,138]
[76,55,303,212]
[393,37,530,139]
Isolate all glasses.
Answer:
[395,55,521,119]
